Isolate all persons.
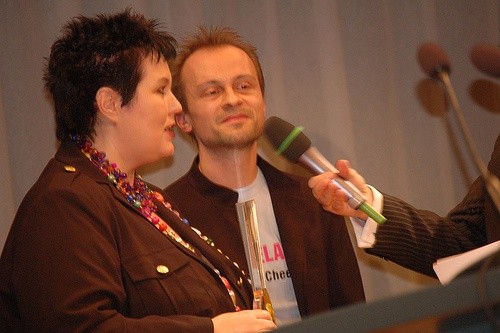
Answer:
[0,8,276,332]
[307,133,500,281]
[164,24,364,324]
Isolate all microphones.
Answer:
[470,43,500,78]
[419,42,500,213]
[264,116,387,225]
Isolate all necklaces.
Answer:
[72,136,253,312]
